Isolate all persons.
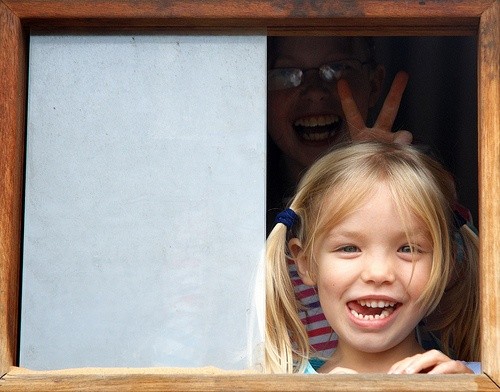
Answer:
[266,37,473,358]
[265,142,479,375]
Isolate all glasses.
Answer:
[267,57,370,92]
